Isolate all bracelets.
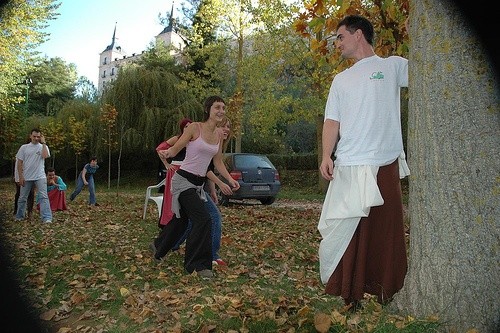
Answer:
[43,142,46,144]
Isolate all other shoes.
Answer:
[149,242,163,263]
[89,202,99,205]
[173,247,186,253]
[212,258,227,266]
[44,220,52,224]
[198,269,214,278]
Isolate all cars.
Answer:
[213,152,281,206]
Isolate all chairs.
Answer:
[143,177,166,220]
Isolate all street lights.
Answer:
[25,78,33,110]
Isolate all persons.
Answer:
[68,157,99,208]
[14,129,53,225]
[36,168,67,213]
[151,96,240,278]
[321,16,410,322]
